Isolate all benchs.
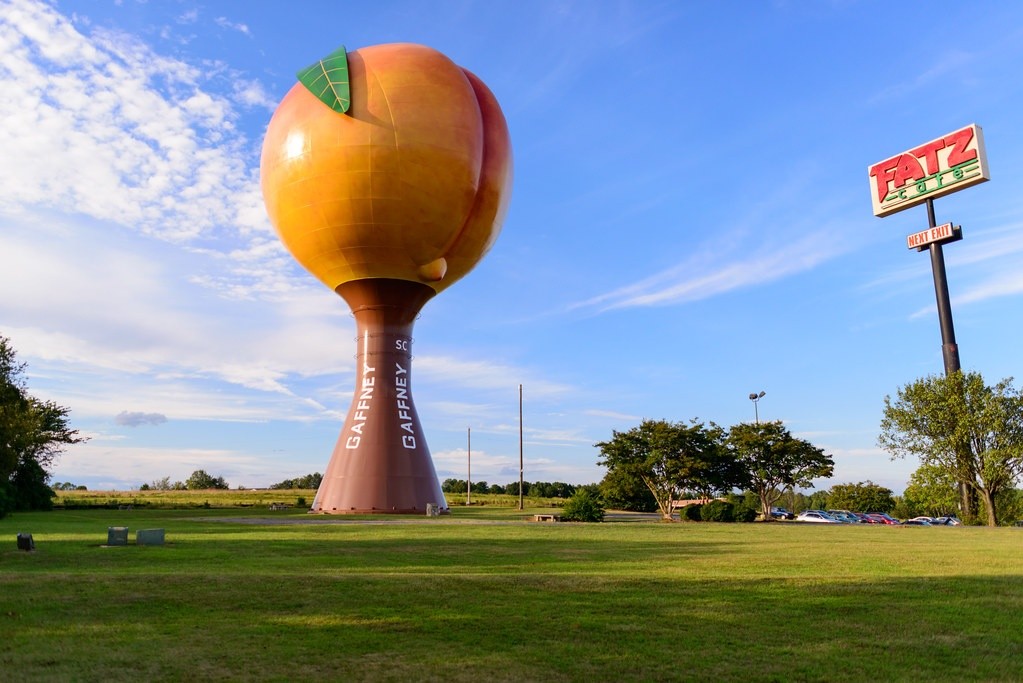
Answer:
[535,515,560,522]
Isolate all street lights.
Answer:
[749,390,766,425]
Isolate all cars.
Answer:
[796,509,963,526]
[770,507,795,520]
